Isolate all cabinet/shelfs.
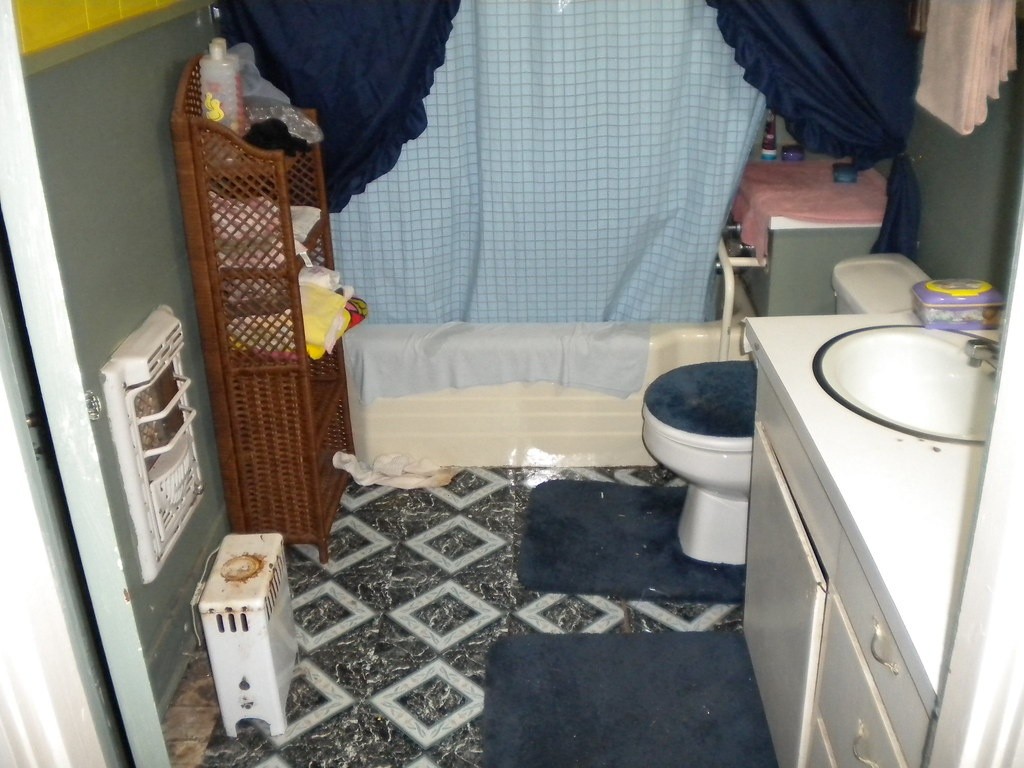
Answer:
[170,56,355,563]
[743,365,933,768]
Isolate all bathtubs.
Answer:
[341,264,755,472]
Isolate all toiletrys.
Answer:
[199,38,251,139]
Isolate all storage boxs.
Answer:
[911,277,1006,330]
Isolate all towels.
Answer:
[221,264,370,360]
[907,0,1019,135]
[331,450,457,490]
[206,189,321,268]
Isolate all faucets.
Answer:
[965,338,1000,372]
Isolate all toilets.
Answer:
[640,252,936,565]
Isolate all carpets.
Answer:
[482,632,778,768]
[517,478,746,603]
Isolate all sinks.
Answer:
[811,325,998,448]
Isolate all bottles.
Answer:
[203,39,238,73]
[199,43,239,135]
